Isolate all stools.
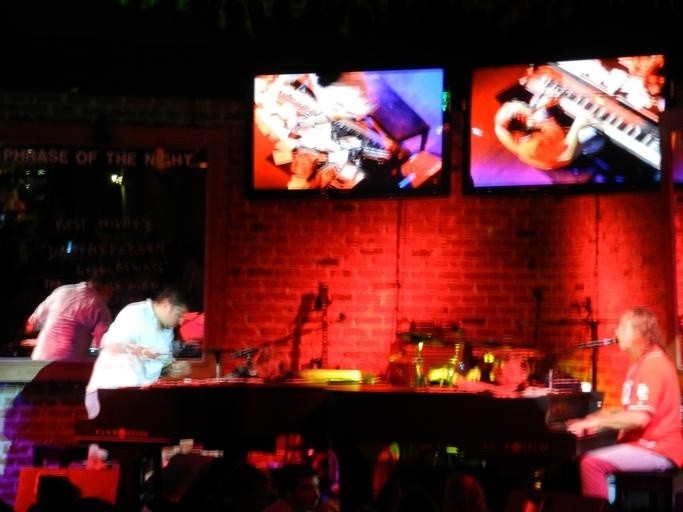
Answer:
[613,469,683,512]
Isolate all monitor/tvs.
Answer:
[241,60,451,200]
[460,41,674,197]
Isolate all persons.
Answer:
[0,419,681,511]
[22,264,116,361]
[566,308,683,500]
[494,87,598,171]
[84,287,192,419]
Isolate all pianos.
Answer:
[276,84,398,168]
[524,62,662,171]
[76,380,620,512]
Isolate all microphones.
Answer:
[170,341,200,353]
[576,336,618,350]
[229,347,259,358]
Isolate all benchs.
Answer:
[32,442,166,511]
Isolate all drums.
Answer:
[490,346,549,384]
[387,345,468,387]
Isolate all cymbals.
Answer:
[397,331,449,346]
